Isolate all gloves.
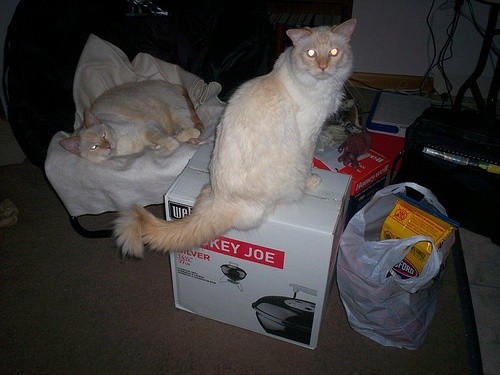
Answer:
[338,132,372,169]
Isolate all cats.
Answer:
[58,17,358,259]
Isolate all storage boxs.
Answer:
[347,177,385,219]
[313,131,404,196]
[163,144,353,351]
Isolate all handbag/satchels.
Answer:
[337,182,455,351]
